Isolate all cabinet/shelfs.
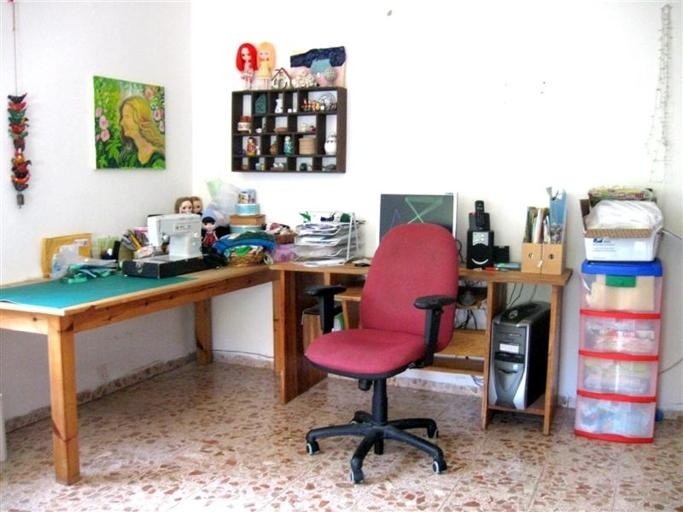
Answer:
[296,211,363,263]
[232,86,347,173]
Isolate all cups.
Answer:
[238,189,255,203]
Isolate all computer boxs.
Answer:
[489,300,551,412]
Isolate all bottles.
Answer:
[273,98,283,114]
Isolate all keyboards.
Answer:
[457,286,488,309]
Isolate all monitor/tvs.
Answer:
[378,192,459,246]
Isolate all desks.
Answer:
[0,256,272,487]
[269,232,574,435]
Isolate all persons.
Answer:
[294,216,359,261]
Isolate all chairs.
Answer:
[299,222,460,484]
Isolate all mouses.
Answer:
[345,256,371,267]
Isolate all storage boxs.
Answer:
[577,198,682,262]
[573,256,663,444]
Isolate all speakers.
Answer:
[467,230,493,270]
